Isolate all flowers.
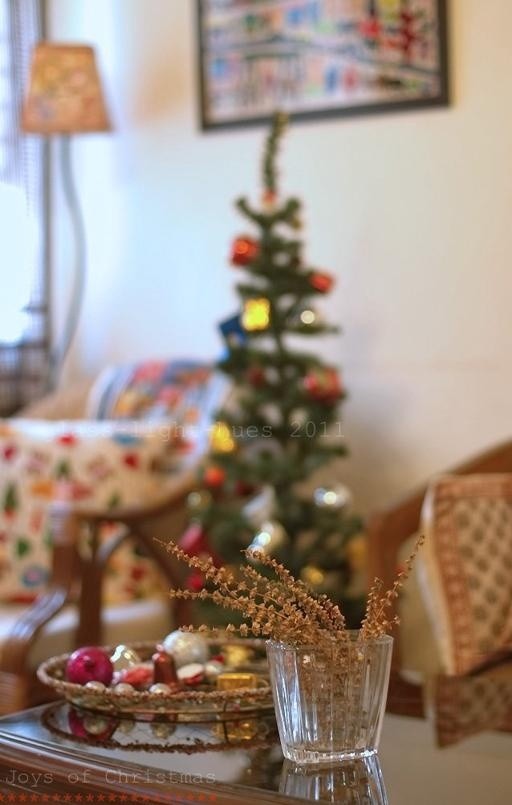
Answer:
[141,524,430,745]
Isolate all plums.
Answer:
[65,646,112,687]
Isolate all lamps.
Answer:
[16,38,119,395]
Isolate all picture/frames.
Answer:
[197,0,451,133]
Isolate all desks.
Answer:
[2,697,512,805]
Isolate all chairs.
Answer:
[0,351,250,721]
[363,434,511,732]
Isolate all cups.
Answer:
[277,758,390,805]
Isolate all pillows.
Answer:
[412,469,509,680]
[0,414,182,612]
[389,523,444,691]
